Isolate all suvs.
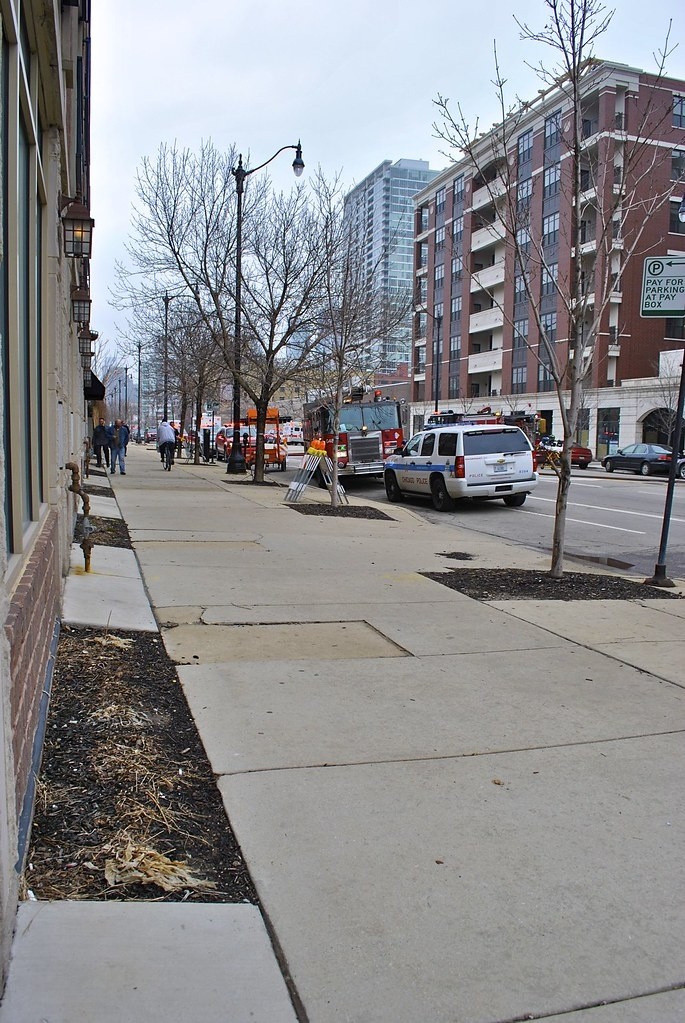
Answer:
[382,423,540,512]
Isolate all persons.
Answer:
[92,418,110,468]
[170,421,180,448]
[105,419,130,475]
[539,434,555,447]
[157,419,175,465]
[122,421,130,456]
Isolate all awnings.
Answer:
[85,370,105,401]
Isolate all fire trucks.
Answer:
[420,406,547,449]
[301,389,405,488]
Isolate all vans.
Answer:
[283,420,304,446]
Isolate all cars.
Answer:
[144,429,157,444]
[600,443,673,476]
[215,426,257,463]
[263,428,283,444]
[176,428,216,459]
[676,449,685,479]
[535,440,593,470]
[129,429,144,441]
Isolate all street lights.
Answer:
[226,138,306,475]
[163,289,201,421]
[136,339,157,443]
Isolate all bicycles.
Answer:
[162,441,173,472]
